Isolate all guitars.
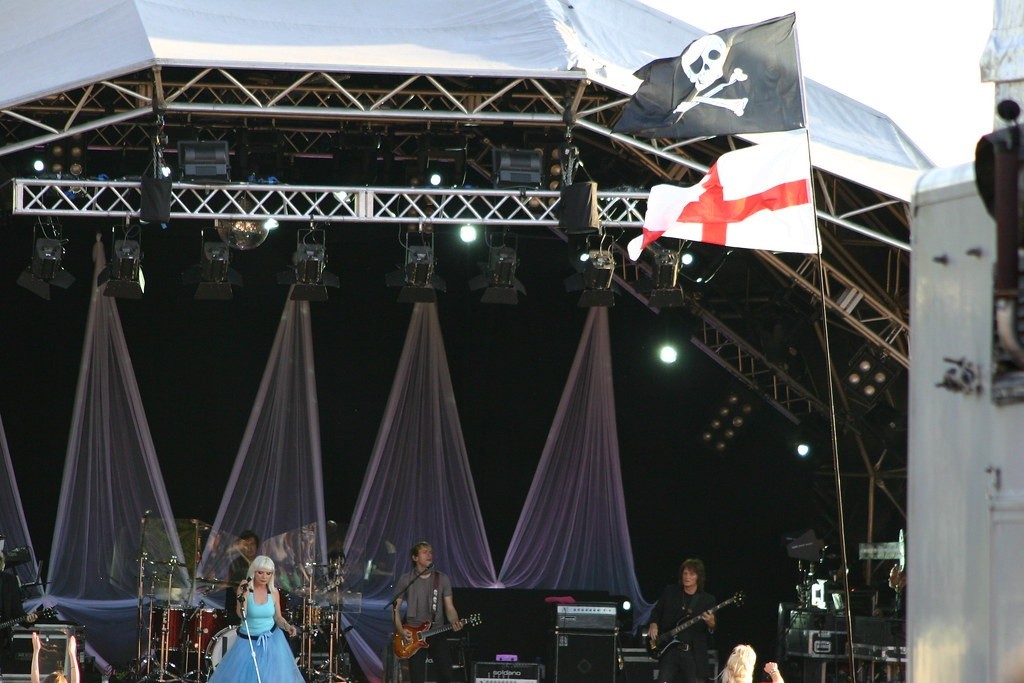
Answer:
[0,605,58,630]
[645,590,745,661]
[393,613,483,661]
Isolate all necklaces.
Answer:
[682,591,685,610]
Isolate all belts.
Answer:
[674,642,692,651]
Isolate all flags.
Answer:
[627,137,824,262]
[612,14,808,136]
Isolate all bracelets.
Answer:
[769,669,776,674]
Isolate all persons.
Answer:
[0,550,38,674]
[206,529,306,683]
[392,541,463,683]
[648,559,719,683]
[709,644,785,683]
[888,563,907,683]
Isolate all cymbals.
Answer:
[143,576,174,583]
[187,577,239,587]
[149,559,191,568]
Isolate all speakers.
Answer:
[560,182,598,233]
[140,178,173,223]
[549,631,618,683]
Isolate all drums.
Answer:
[181,607,229,654]
[295,604,322,627]
[203,625,242,676]
[149,604,193,652]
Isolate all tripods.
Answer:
[121,512,207,683]
[295,565,351,683]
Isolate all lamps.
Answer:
[217,194,270,251]
[394,230,439,303]
[474,246,523,303]
[490,145,543,192]
[649,250,691,310]
[175,139,232,183]
[98,231,149,298]
[289,238,331,299]
[192,239,233,302]
[577,251,617,308]
[17,235,76,302]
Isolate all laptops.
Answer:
[22,560,44,585]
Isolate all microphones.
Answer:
[428,563,434,568]
[240,576,253,590]
[146,509,152,513]
